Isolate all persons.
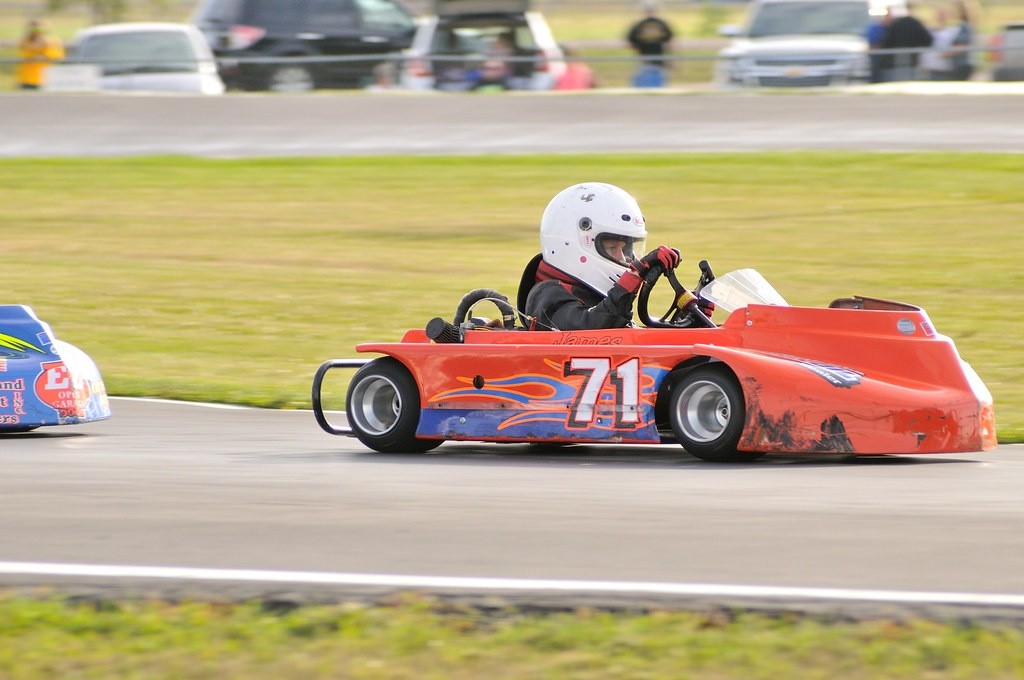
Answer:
[864,0,980,81]
[451,32,518,81]
[625,2,674,66]
[522,180,713,331]
[14,20,66,89]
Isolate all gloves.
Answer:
[630,246,682,281]
[691,291,715,319]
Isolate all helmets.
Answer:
[539,182,647,298]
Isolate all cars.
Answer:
[43,22,227,93]
[192,0,419,91]
[715,1,910,88]
[401,0,567,91]
